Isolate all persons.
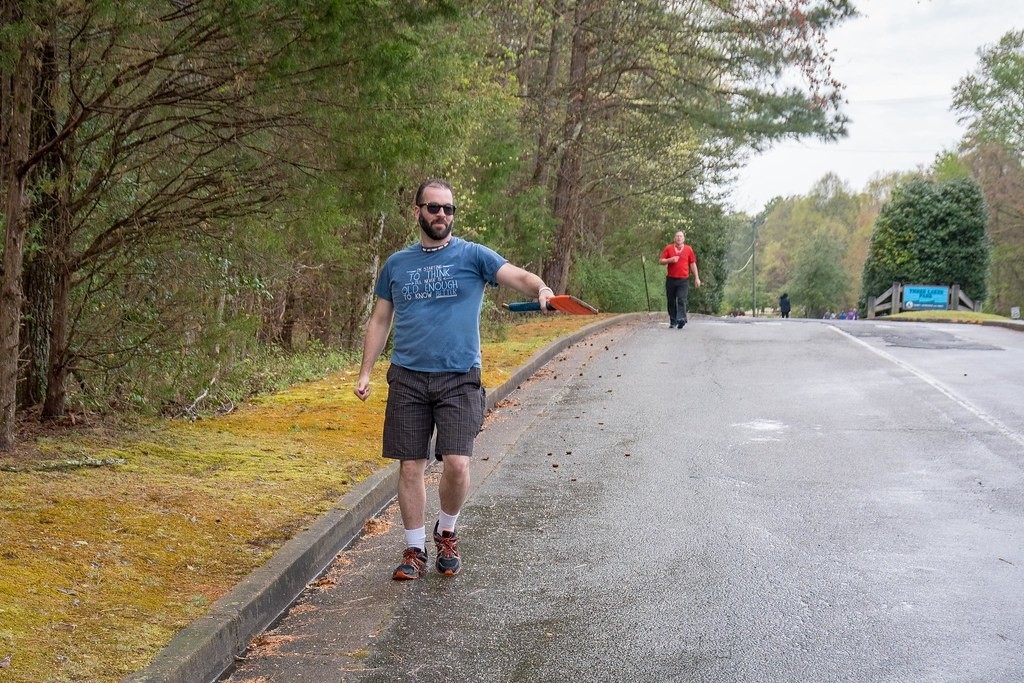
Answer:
[822,307,858,320]
[779,293,791,318]
[352,178,558,581]
[658,231,701,329]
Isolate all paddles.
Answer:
[500,294,600,317]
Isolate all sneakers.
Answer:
[393,546,428,579]
[433,519,461,576]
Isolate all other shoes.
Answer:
[670,323,677,329]
[678,318,685,329]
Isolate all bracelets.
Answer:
[539,287,553,295]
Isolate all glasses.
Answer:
[417,202,456,216]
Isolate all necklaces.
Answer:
[421,240,449,252]
[675,244,684,253]
